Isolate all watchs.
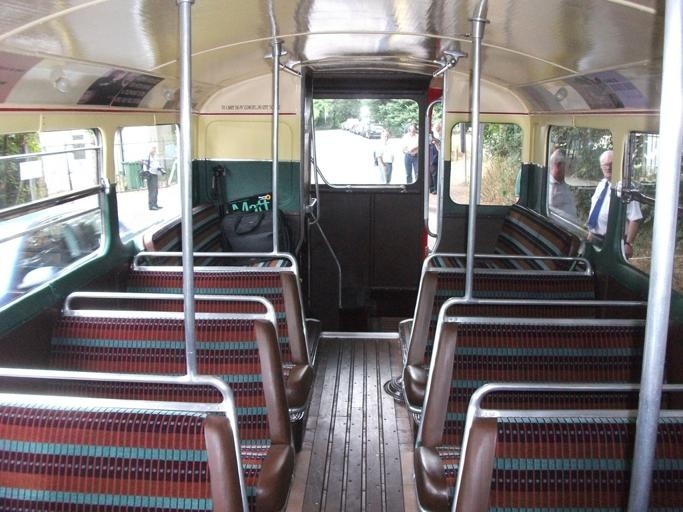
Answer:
[625,242,634,248]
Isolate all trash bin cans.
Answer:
[123,161,144,190]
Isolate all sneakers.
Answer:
[150,205,161,209]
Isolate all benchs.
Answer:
[428,203,587,270]
[401,252,682,508]
[133,202,293,267]
[1,250,324,508]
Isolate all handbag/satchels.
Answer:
[140,171,151,179]
[220,209,291,264]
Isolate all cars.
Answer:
[0,207,130,308]
[342,119,384,139]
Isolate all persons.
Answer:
[548,149,578,221]
[428,123,443,194]
[376,131,395,184]
[401,125,419,184]
[143,148,167,210]
[585,151,644,262]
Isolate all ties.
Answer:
[585,182,609,228]
